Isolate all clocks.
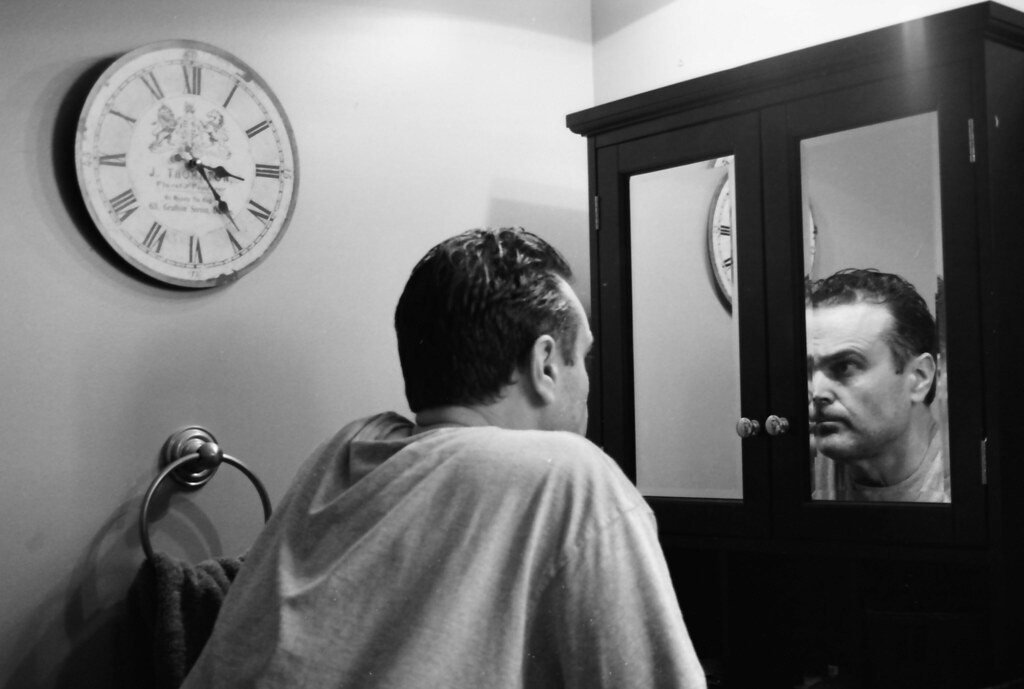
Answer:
[705,177,732,313]
[75,37,301,288]
[802,199,822,275]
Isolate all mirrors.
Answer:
[623,151,747,505]
[799,108,954,506]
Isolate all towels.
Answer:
[125,551,253,689]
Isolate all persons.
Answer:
[805,271,950,502]
[182,227,708,689]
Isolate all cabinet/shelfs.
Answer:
[565,0,1024,689]
